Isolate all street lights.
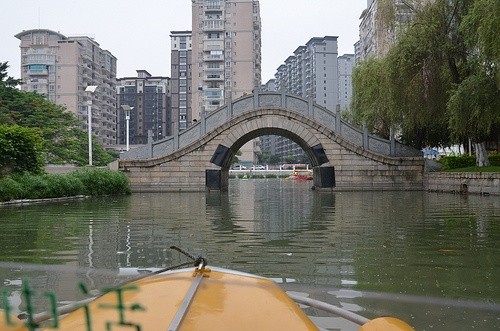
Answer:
[85,85,98,166]
[120,104,135,151]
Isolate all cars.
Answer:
[231,164,247,170]
[250,164,265,170]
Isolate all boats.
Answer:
[0,258,417,331]
